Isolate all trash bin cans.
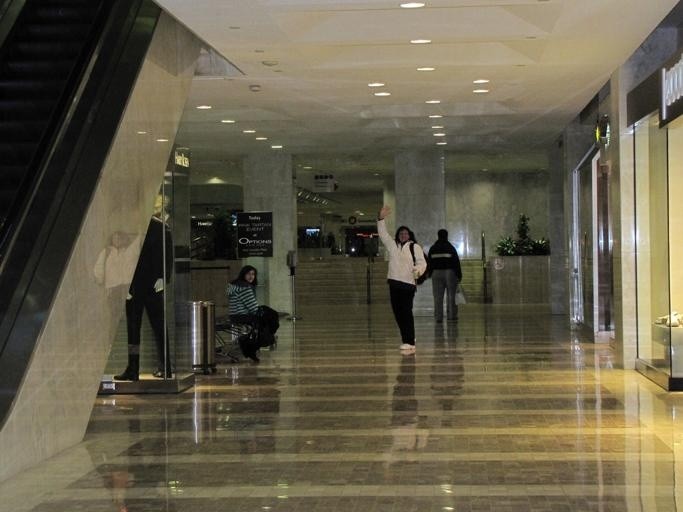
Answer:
[188,300,218,375]
[192,375,219,446]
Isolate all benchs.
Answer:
[215,312,289,364]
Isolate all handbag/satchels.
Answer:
[455,280,468,306]
[239,321,258,360]
[409,243,432,285]
[255,306,279,333]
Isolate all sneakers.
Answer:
[400,344,415,350]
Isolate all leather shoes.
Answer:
[113,370,140,382]
[151,369,172,379]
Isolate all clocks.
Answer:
[348,216,356,224]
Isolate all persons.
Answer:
[426,227,462,322]
[113,214,172,380]
[375,204,427,351]
[388,337,470,476]
[223,264,280,364]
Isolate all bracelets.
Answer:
[378,217,383,222]
